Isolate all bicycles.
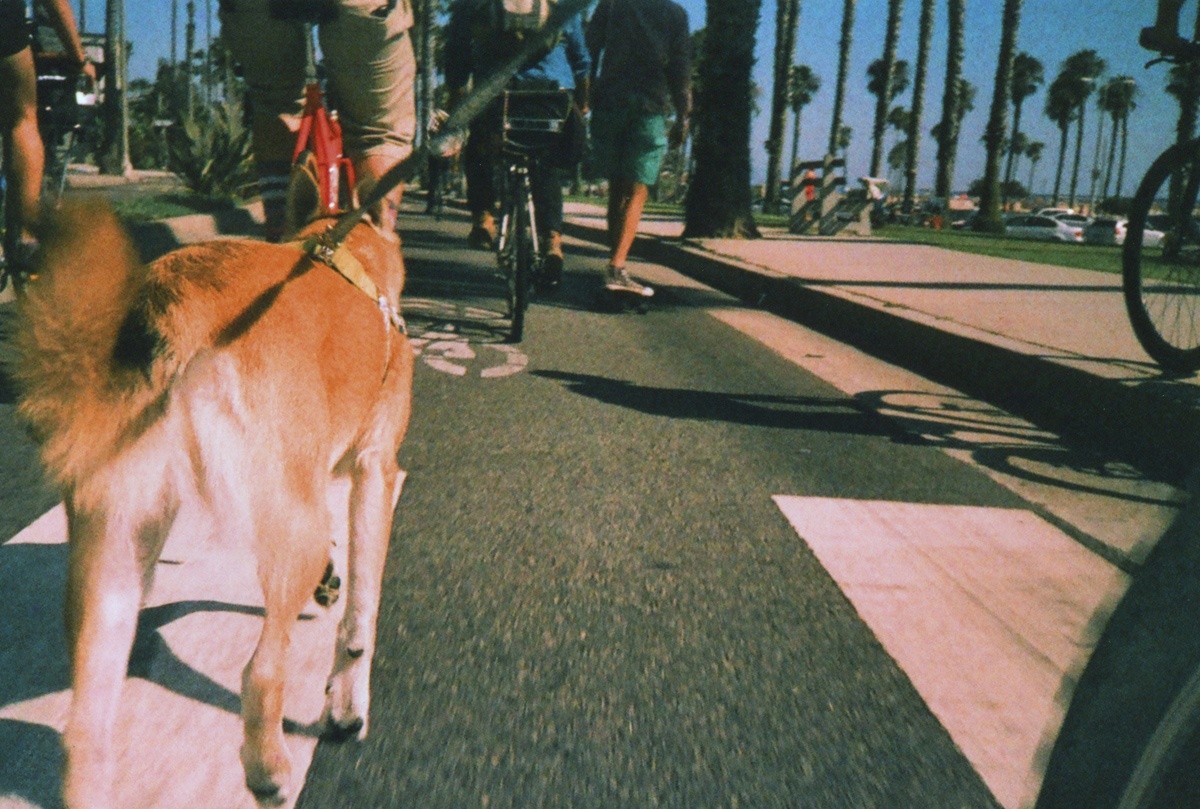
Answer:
[462,77,587,345]
[1121,29,1200,366]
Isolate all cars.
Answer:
[1032,206,1096,229]
[1005,214,1086,246]
[1084,218,1167,248]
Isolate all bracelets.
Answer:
[78,52,89,69]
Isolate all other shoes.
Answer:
[471,212,496,249]
[537,250,564,294]
[605,264,653,297]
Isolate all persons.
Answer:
[215,0,418,231]
[427,0,590,279]
[583,0,689,296]
[0,0,96,287]
[857,176,891,209]
[804,167,816,205]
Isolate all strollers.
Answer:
[22,19,134,211]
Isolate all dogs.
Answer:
[1,143,416,809]
